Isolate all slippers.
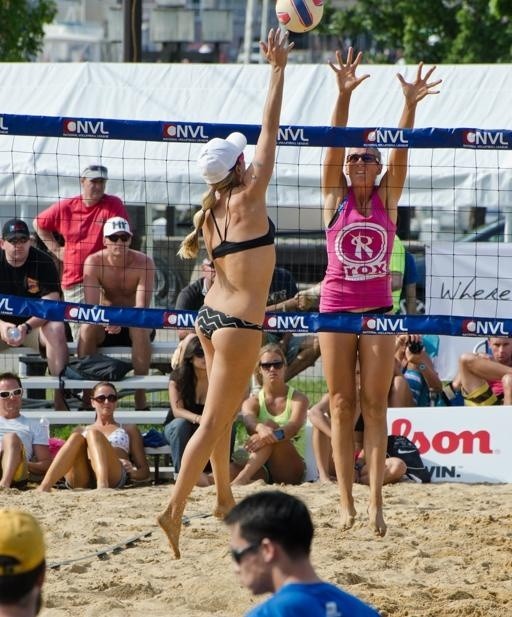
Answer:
[387,435,431,484]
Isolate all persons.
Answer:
[0,507,46,617]
[319,47,442,538]
[164,335,309,483]
[176,257,216,340]
[386,252,511,407]
[32,164,128,343]
[0,373,51,490]
[0,218,70,411]
[36,382,149,491]
[158,27,295,560]
[255,236,406,386]
[308,347,408,486]
[223,491,381,617]
[78,217,156,410]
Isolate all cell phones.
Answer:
[408,341,424,355]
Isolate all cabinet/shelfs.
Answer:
[8,328,21,338]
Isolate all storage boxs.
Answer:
[17,348,175,484]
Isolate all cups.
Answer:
[91,394,118,404]
[81,165,107,174]
[260,362,284,369]
[346,154,379,164]
[8,237,30,244]
[0,388,23,400]
[195,348,205,358]
[106,234,131,242]
[206,261,214,268]
[232,542,261,566]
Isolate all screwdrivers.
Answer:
[276,0,325,34]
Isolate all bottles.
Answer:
[2,220,29,242]
[0,507,46,578]
[80,168,108,180]
[197,131,248,186]
[103,217,133,237]
